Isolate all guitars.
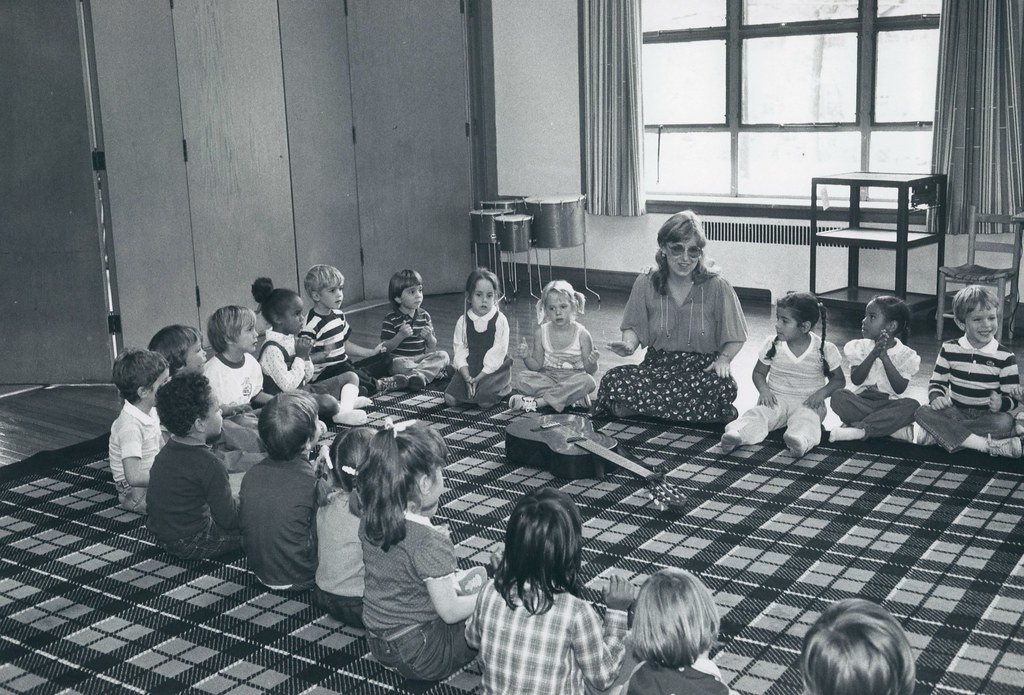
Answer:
[505,411,690,507]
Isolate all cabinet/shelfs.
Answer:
[810,172,947,347]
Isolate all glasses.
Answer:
[669,245,701,258]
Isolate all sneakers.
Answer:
[572,396,591,409]
[986,435,1022,459]
[509,394,538,413]
[378,373,409,393]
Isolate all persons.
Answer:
[596,211,749,423]
[829,295,922,443]
[148,324,270,472]
[315,427,379,630]
[381,270,454,391]
[914,285,1024,458]
[510,280,600,412]
[444,267,511,407]
[722,291,847,455]
[202,307,276,415]
[800,599,915,695]
[622,568,740,695]
[250,277,373,425]
[238,392,320,591]
[299,265,407,396]
[465,488,636,695]
[145,372,239,561]
[354,426,504,681]
[108,349,246,516]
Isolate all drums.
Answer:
[524,194,585,250]
[494,216,533,253]
[468,210,516,245]
[480,196,528,216]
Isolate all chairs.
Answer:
[936,206,1024,342]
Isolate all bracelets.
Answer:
[722,353,729,357]
[464,376,471,383]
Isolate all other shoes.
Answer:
[411,376,425,392]
[455,566,488,595]
